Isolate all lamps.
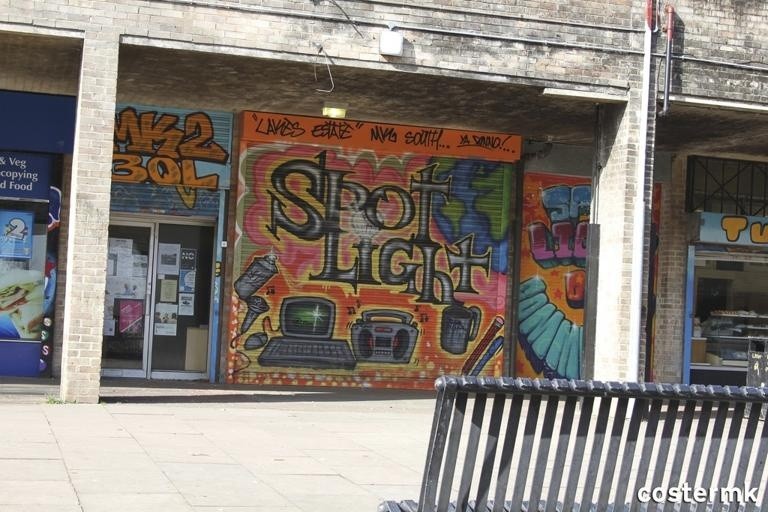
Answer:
[377,22,404,57]
[320,100,349,120]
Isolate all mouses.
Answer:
[243,332,267,352]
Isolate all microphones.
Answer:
[240,295,269,336]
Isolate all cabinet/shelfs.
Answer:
[691,337,706,364]
[701,311,766,368]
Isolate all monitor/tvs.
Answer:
[279,295,337,340]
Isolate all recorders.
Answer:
[351,308,419,364]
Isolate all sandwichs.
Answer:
[0,268,45,339]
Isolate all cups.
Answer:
[440,303,482,355]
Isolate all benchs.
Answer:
[380,374,768,512]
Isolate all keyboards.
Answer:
[258,337,357,371]
